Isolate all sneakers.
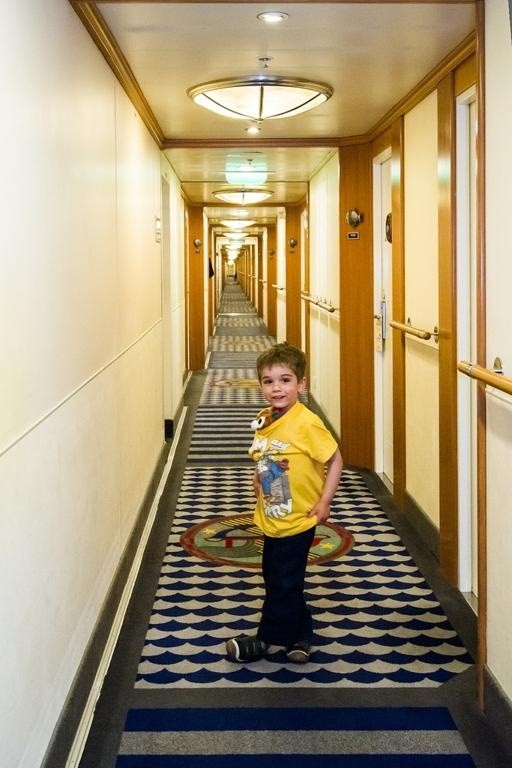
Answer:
[287,638,310,663]
[227,635,270,663]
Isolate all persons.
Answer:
[226,341,344,664]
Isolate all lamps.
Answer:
[218,219,257,266]
[213,187,274,207]
[186,75,334,125]
